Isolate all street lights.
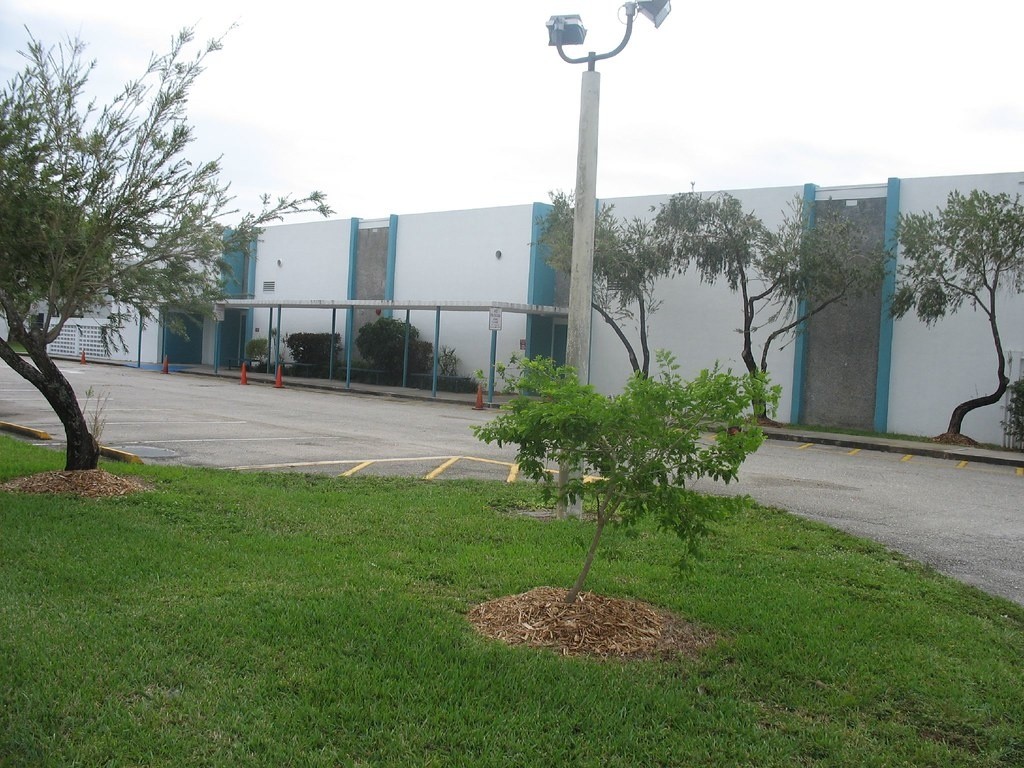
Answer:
[545,0,672,520]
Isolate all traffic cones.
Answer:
[727,426,741,435]
[238,362,250,385]
[79,350,87,364]
[160,355,170,374]
[471,381,486,410]
[272,364,284,388]
